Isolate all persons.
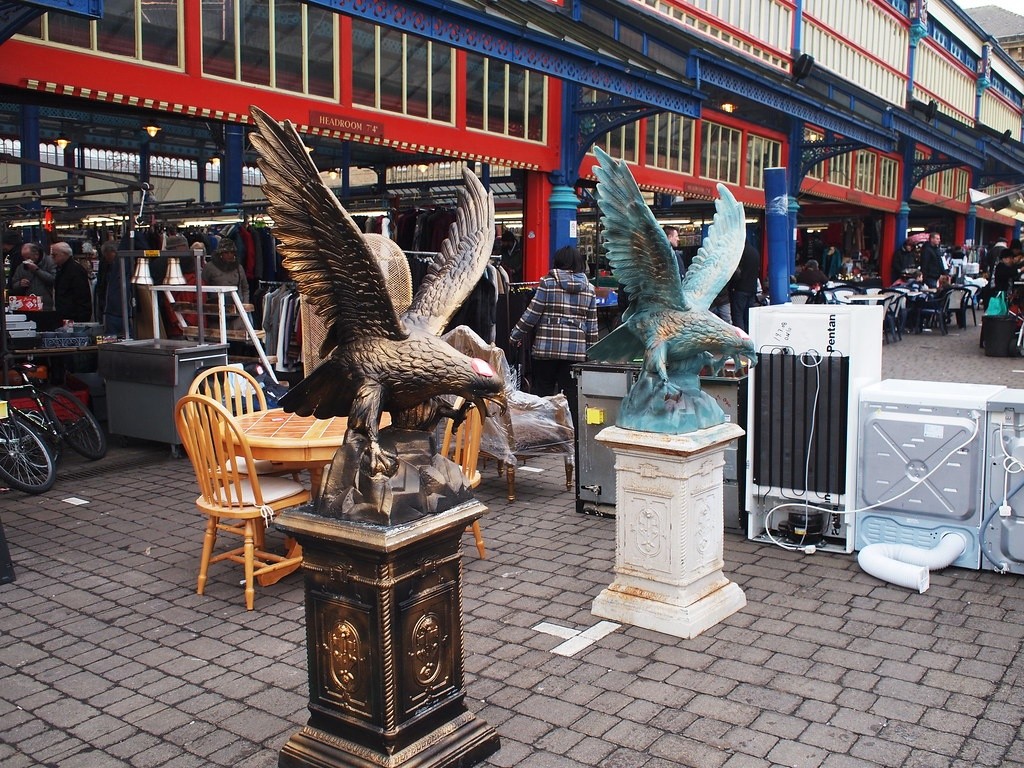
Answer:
[1,230,253,356]
[822,246,842,279]
[797,260,827,304]
[664,226,685,281]
[511,245,598,421]
[709,285,732,325]
[893,233,950,326]
[981,237,1024,350]
[728,238,760,334]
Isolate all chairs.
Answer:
[174,392,309,612]
[443,325,575,506]
[189,365,305,550]
[441,395,486,561]
[789,280,983,344]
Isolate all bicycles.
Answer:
[0,352,108,493]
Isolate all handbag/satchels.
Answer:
[985,291,1009,316]
[205,296,240,322]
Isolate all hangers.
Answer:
[85,223,213,236]
[410,252,435,263]
[259,281,296,292]
[346,201,451,219]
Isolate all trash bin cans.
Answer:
[982,314,1018,357]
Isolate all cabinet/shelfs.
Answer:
[96,249,231,459]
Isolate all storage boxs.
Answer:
[7,337,38,350]
[37,332,96,348]
[8,330,37,338]
[5,314,27,321]
[6,321,36,330]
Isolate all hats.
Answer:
[215,238,237,253]
[1010,239,1022,250]
[999,249,1014,258]
[166,236,187,248]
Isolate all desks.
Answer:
[908,292,923,298]
[845,295,893,304]
[219,407,391,501]
[922,288,937,298]
[979,314,1019,358]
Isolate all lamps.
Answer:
[162,257,189,287]
[130,258,154,286]
[790,53,815,85]
[924,98,939,125]
[999,129,1012,146]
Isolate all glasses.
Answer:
[50,252,59,258]
[223,251,234,254]
